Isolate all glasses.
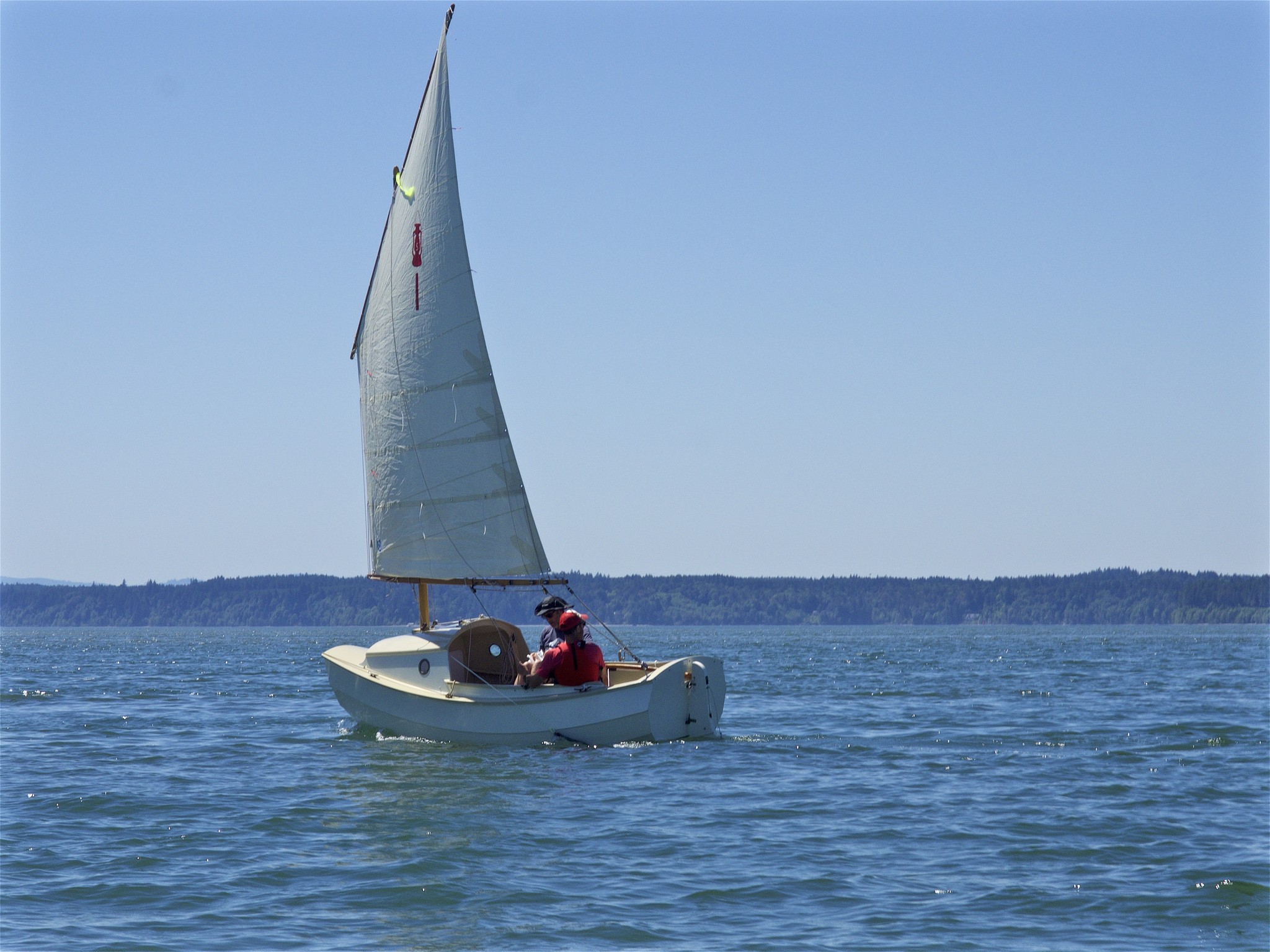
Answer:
[541,610,556,620]
[575,620,586,629]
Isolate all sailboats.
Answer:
[319,3,730,746]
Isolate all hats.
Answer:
[559,609,588,631]
[534,595,574,616]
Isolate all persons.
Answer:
[513,596,592,686]
[531,609,606,685]
